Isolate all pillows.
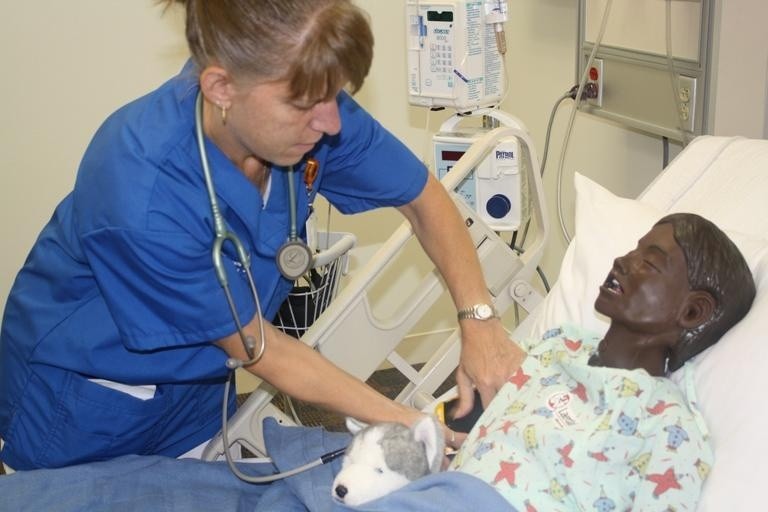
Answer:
[513,171,768,349]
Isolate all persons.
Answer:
[454,212,758,512]
[0,0,518,473]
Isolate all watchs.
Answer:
[457,303,501,320]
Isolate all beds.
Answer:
[198,123,768,512]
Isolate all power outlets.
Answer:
[581,58,603,108]
